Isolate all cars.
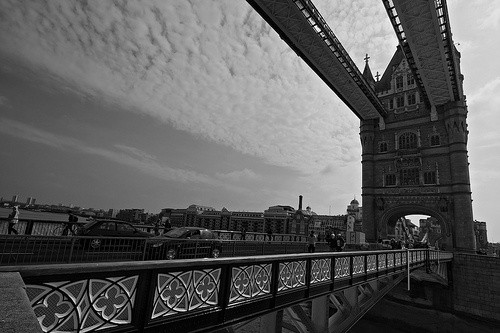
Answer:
[147,227,223,260]
[81,219,156,250]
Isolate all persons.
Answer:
[336,233,345,245]
[155,219,161,236]
[325,233,332,242]
[163,220,171,234]
[307,230,316,252]
[8,205,20,234]
[65,210,77,236]
